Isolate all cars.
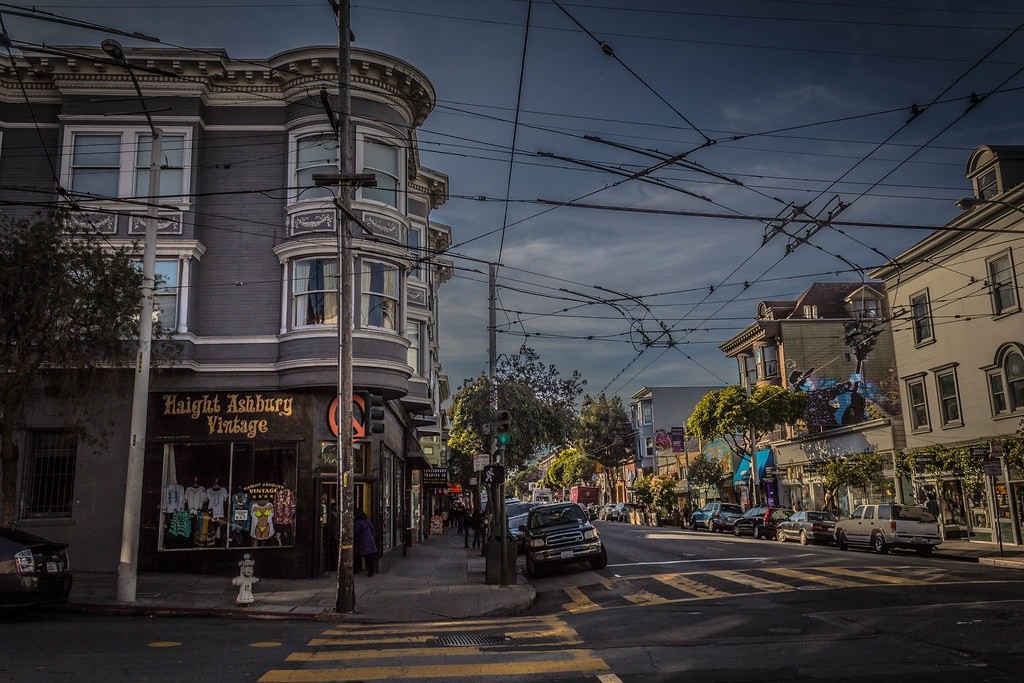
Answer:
[507,513,528,551]
[0,526,74,620]
[775,510,842,545]
[733,507,795,539]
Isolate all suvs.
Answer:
[611,502,641,522]
[832,503,943,556]
[518,502,607,576]
[690,501,743,533]
[598,503,615,521]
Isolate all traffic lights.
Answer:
[365,392,385,435]
[493,410,511,447]
[483,465,505,484]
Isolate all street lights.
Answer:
[102,39,165,604]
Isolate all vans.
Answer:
[504,502,538,520]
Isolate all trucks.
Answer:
[570,486,600,512]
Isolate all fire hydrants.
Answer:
[231,554,260,607]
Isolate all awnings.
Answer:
[733,449,772,485]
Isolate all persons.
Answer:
[354,509,377,577]
[449,501,485,549]
[925,493,940,518]
[587,502,696,528]
[793,501,803,512]
